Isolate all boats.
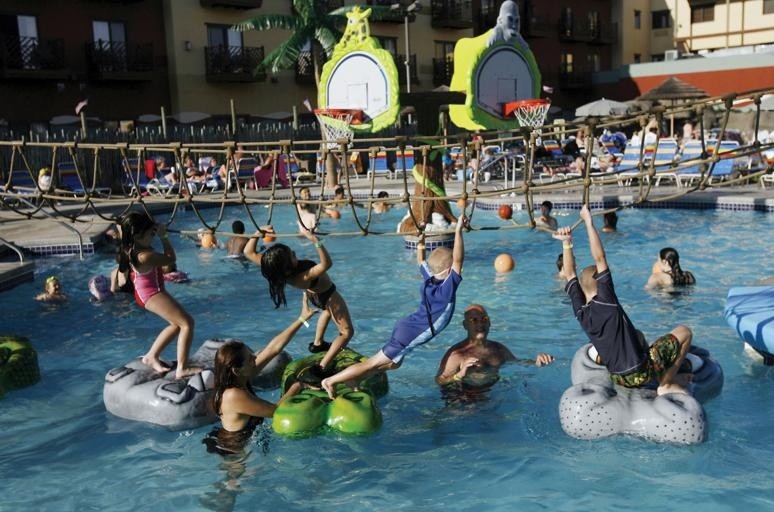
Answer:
[719,281,774,356]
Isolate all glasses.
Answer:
[469,316,489,323]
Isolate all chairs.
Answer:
[120,152,316,199]
[1,162,112,209]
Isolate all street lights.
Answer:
[388,1,424,124]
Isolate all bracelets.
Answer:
[297,316,310,328]
[453,373,461,382]
[416,245,426,250]
[314,238,326,248]
[562,243,574,250]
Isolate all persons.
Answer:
[210,291,322,456]
[551,206,695,398]
[555,254,579,279]
[645,247,696,296]
[38,116,773,204]
[600,211,618,233]
[224,220,249,255]
[511,200,557,228]
[485,1,528,49]
[121,212,204,379]
[200,228,218,249]
[36,215,186,304]
[244,224,355,378]
[350,191,408,214]
[434,304,556,403]
[320,216,469,399]
[296,187,324,233]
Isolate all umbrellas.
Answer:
[710,93,774,113]
[574,97,627,117]
[636,77,711,137]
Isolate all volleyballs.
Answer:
[201,234,216,248]
[495,254,513,273]
[499,205,512,219]
[331,210,340,219]
[263,230,275,243]
[458,199,468,209]
[652,262,662,273]
